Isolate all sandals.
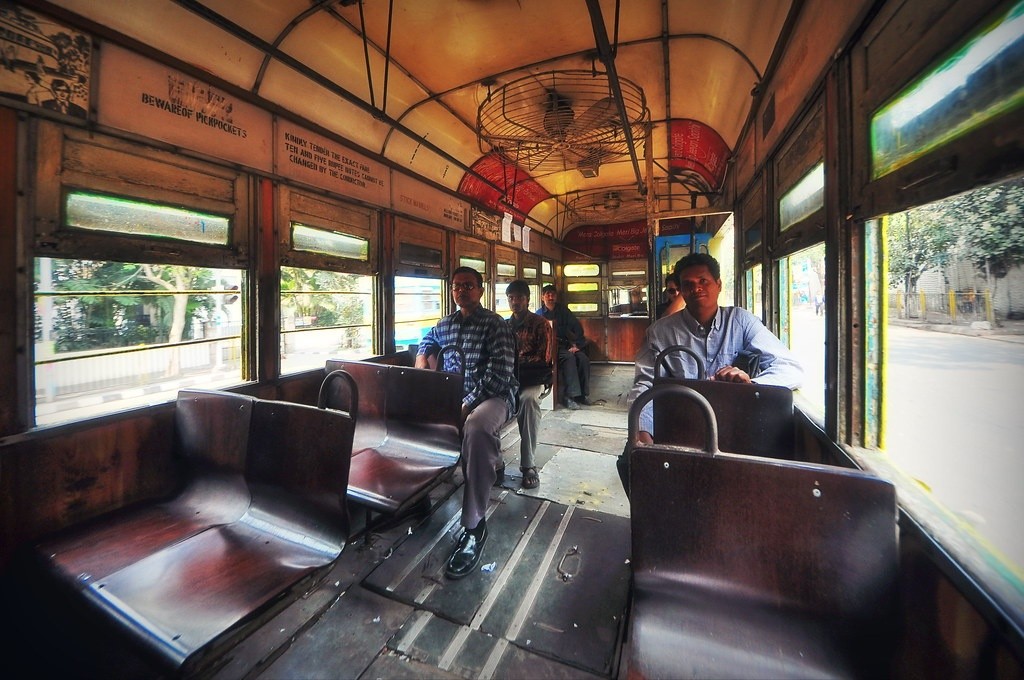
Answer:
[521,465,540,489]
[493,467,506,487]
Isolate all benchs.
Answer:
[34,319,915,680]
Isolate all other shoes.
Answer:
[577,395,593,405]
[563,398,580,410]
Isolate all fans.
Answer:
[564,191,646,226]
[478,66,650,171]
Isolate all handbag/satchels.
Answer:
[730,350,760,378]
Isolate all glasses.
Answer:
[449,282,481,291]
[667,287,680,294]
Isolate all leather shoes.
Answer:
[446,525,489,576]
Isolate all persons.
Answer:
[414,267,520,580]
[656,272,687,319]
[534,285,592,409]
[616,252,805,500]
[502,279,553,488]
